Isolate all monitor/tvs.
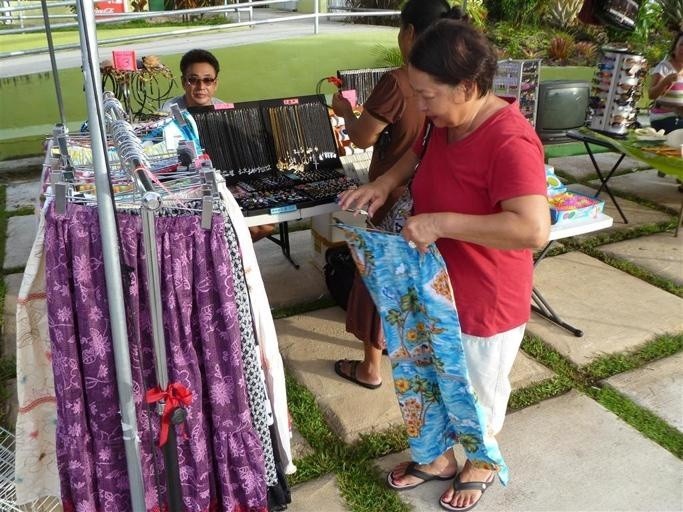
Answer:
[536,80,592,145]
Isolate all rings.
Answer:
[228,169,359,210]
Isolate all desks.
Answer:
[531,213,614,337]
[565,127,683,224]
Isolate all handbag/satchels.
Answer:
[380,187,415,233]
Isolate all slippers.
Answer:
[334,359,382,390]
[386,460,457,491]
[440,472,495,512]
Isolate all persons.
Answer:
[337,21,551,511]
[331,2,458,388]
[157,49,273,243]
[649,31,682,191]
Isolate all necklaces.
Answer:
[315,76,342,109]
[339,68,387,105]
[207,105,338,177]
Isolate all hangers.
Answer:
[40,90,227,230]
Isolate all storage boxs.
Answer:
[311,210,369,274]
